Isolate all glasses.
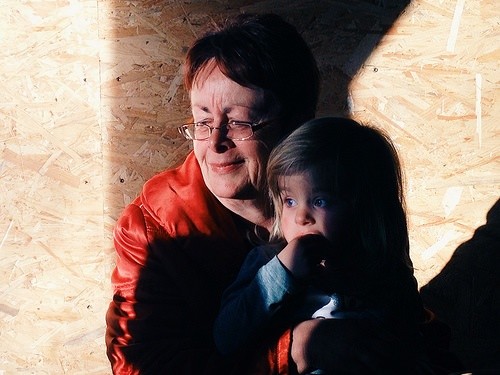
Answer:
[182,120,269,141]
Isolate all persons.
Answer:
[213,115,430,375]
[106,13,321,375]
